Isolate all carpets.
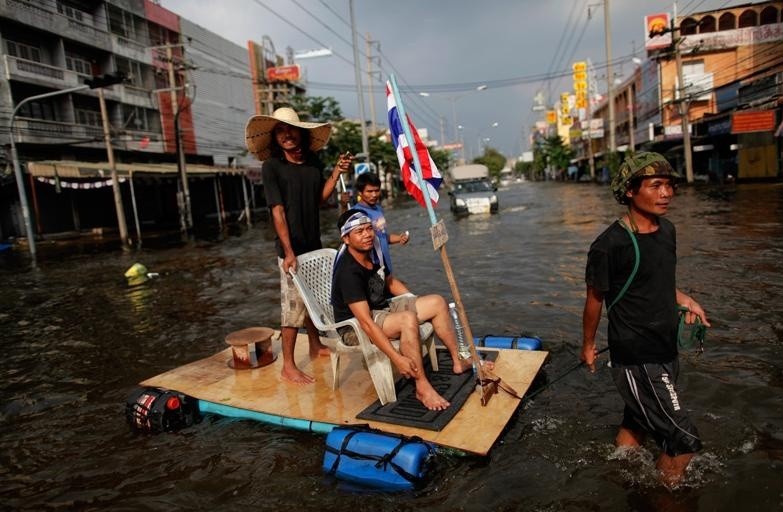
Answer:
[354,347,498,432]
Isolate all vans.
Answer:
[449,164,490,191]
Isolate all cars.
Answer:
[447,178,502,220]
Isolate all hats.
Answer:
[611,152,680,205]
[245,107,331,161]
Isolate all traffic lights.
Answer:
[82,67,127,89]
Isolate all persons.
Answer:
[580,151,711,482]
[348,175,409,297]
[330,209,472,411]
[262,122,354,387]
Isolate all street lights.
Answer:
[456,121,498,155]
[418,84,488,143]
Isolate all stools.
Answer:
[223,327,278,371]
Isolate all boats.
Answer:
[125,328,549,491]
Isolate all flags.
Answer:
[385,75,443,209]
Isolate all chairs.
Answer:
[285,247,438,406]
